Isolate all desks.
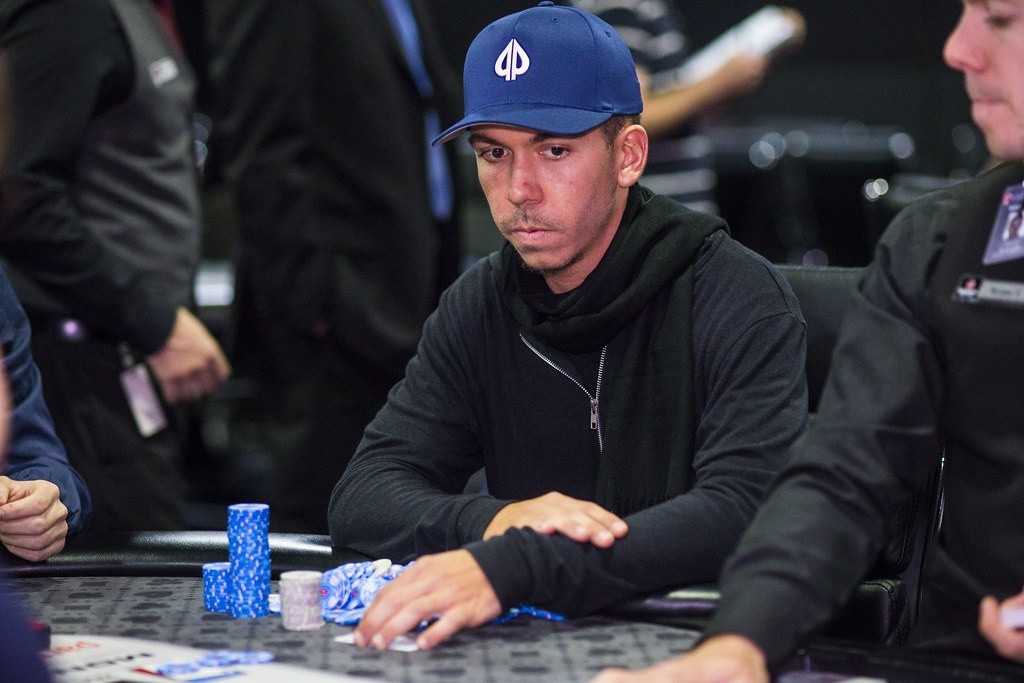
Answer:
[2,557,707,683]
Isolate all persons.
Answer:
[329,0,810,649]
[570,0,767,222]
[215,0,473,522]
[0,0,232,524]
[588,0,1024,683]
[1,268,91,682]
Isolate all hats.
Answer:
[426,0,643,150]
